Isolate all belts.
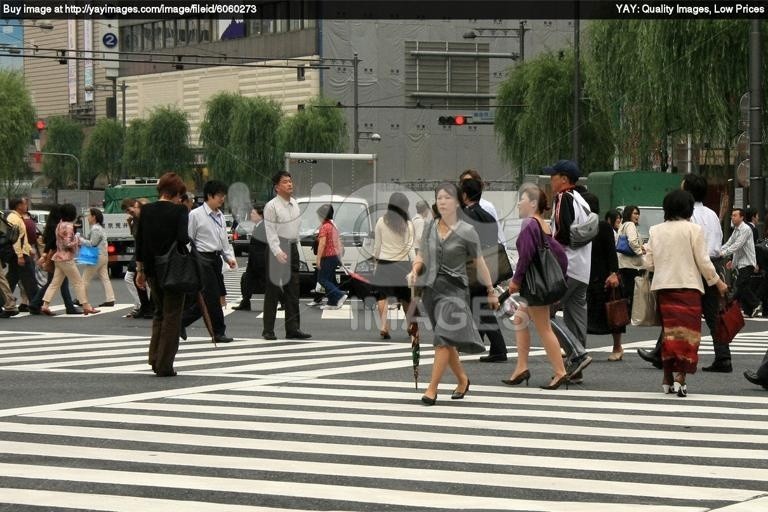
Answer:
[211,250,220,255]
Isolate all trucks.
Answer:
[81,177,161,278]
[519,174,588,222]
[281,151,378,296]
[585,172,689,250]
[190,195,225,218]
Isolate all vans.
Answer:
[27,209,50,236]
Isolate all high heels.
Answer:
[422,378,470,405]
[502,353,570,390]
[662,380,688,397]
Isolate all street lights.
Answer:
[1,23,55,60]
[353,131,381,152]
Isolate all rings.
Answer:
[498,303,500,306]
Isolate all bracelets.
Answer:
[486,287,497,295]
[135,265,144,272]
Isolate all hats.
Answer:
[542,159,581,177]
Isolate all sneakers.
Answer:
[0,299,114,318]
[306,294,348,310]
[564,354,592,383]
[126,308,152,318]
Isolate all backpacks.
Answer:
[1,218,19,244]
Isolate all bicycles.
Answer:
[0,212,48,287]
[701,254,737,319]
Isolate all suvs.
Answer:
[234,222,256,256]
[224,221,234,243]
[233,201,265,228]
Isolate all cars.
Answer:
[753,240,768,270]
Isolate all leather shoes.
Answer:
[743,368,768,389]
[263,332,277,340]
[637,347,664,369]
[702,358,732,372]
[212,335,233,342]
[480,353,507,362]
[608,348,624,361]
[231,304,251,310]
[286,330,312,339]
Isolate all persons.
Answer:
[641,189,728,397]
[637,172,732,374]
[1,162,767,342]
[133,171,189,378]
[501,187,571,390]
[408,180,498,403]
[587,220,629,364]
[460,180,508,363]
[535,160,595,384]
[743,350,767,389]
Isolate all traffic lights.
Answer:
[34,119,49,131]
[438,115,464,126]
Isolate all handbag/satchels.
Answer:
[497,243,513,281]
[714,298,745,345]
[616,235,639,256]
[604,297,630,330]
[79,245,99,266]
[551,211,600,249]
[629,276,658,327]
[24,219,36,244]
[154,245,200,296]
[524,247,569,305]
